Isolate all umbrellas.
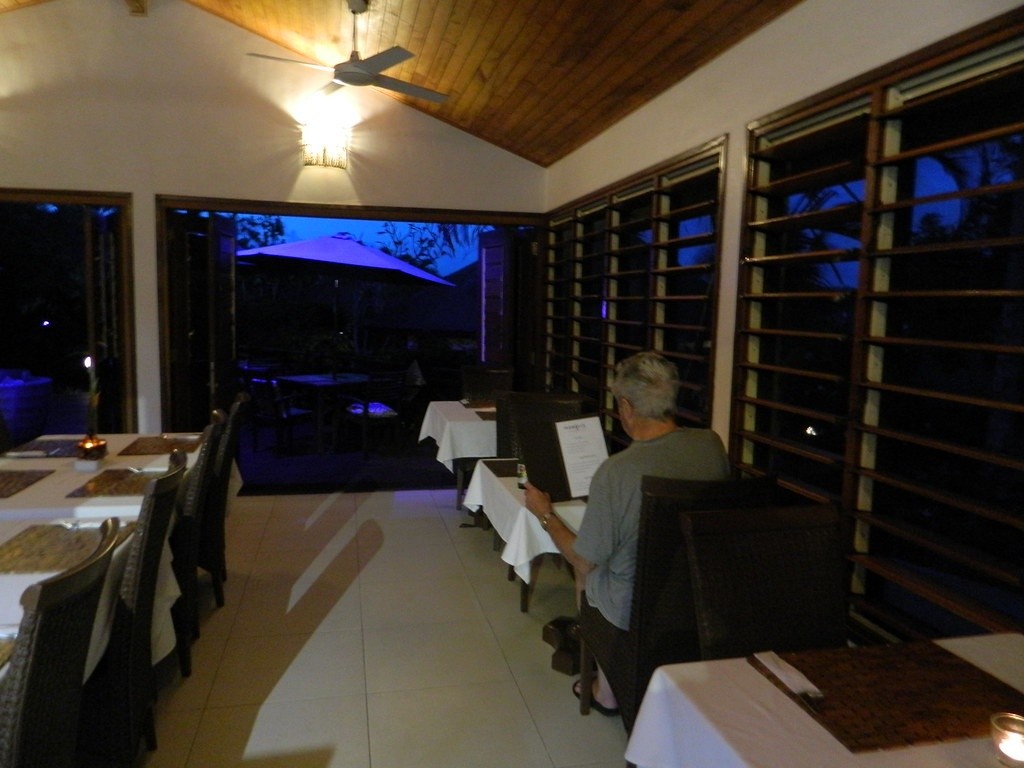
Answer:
[236,231,458,381]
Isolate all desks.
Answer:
[461,456,588,613]
[419,400,497,510]
[0,432,243,687]
[276,372,369,457]
[623,633,1024,768]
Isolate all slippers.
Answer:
[571,677,623,717]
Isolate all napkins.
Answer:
[754,650,820,695]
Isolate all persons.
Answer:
[525,352,730,716]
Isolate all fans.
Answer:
[245,0,450,105]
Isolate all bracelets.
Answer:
[540,512,557,531]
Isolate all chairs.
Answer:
[343,367,409,456]
[678,498,849,659]
[482,391,584,529]
[155,407,229,676]
[75,448,189,768]
[0,516,121,768]
[460,366,514,488]
[580,471,779,732]
[197,391,252,608]
[493,394,582,551]
[243,366,312,456]
